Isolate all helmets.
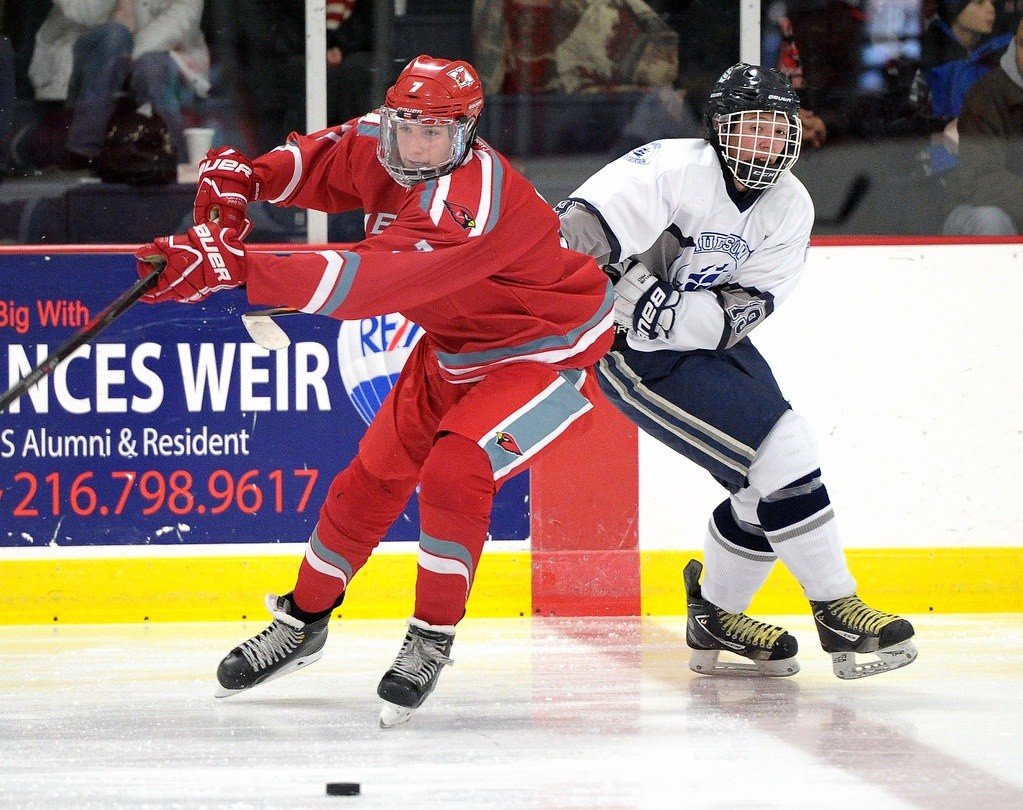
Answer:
[704,62,803,189]
[376,55,484,187]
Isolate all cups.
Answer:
[182,128,215,165]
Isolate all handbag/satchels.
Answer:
[93,108,177,187]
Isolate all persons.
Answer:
[553,59,918,680]
[130,54,616,731]
[0,1,1022,250]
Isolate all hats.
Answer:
[935,0,971,28]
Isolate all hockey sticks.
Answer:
[0,251,167,408]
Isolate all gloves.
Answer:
[602,256,682,341]
[193,145,263,232]
[134,215,255,305]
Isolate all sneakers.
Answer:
[377,606,466,728]
[809,592,918,680]
[683,558,801,677]
[214,590,346,698]
